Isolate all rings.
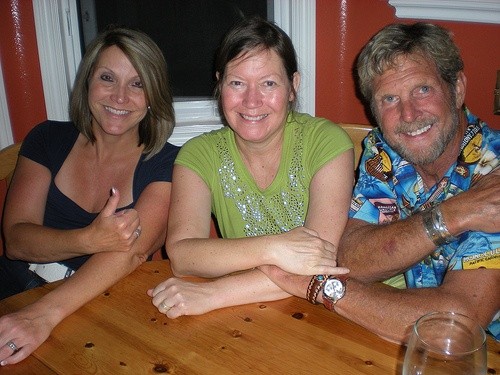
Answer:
[7,341,19,352]
[136,229,141,238]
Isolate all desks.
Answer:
[0,260,500,375]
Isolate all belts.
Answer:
[29,260,76,284]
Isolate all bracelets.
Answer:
[423,207,461,246]
[306,275,330,305]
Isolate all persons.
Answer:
[257,22,500,360]
[0,29,178,366]
[147,22,354,319]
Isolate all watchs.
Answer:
[323,275,352,311]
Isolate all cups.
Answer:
[402,312,488,375]
